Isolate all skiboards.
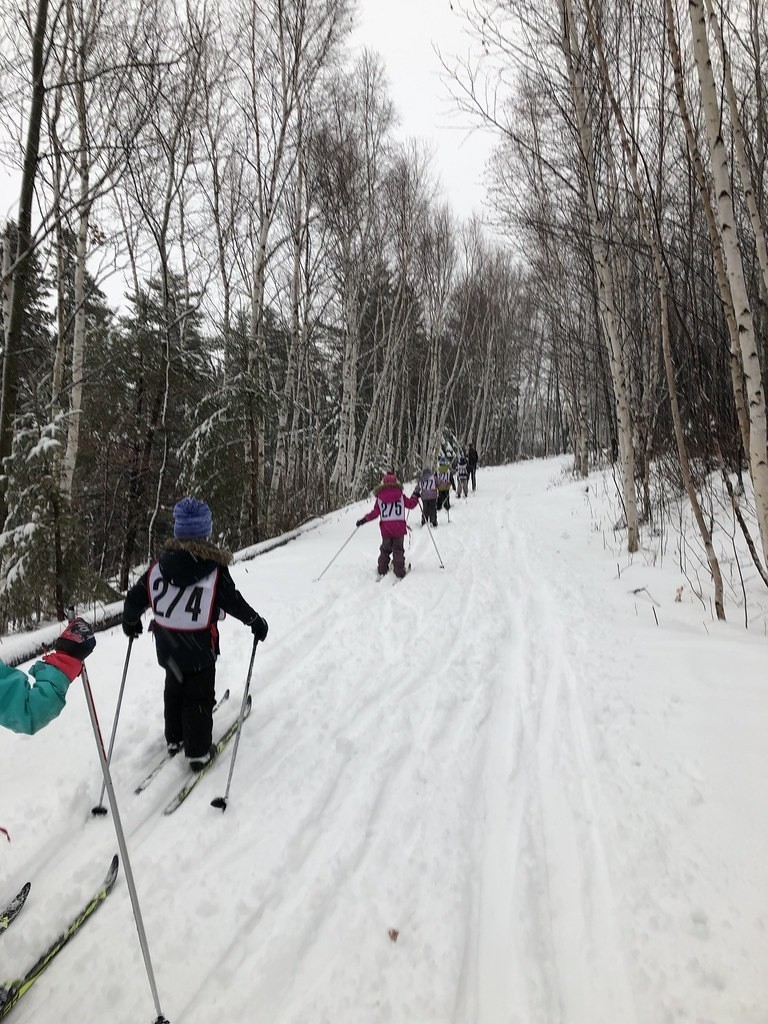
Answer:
[131,686,254,815]
[0,852,120,1023]
[375,549,414,586]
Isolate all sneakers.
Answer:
[167,739,183,755]
[190,742,217,771]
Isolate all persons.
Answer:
[434,457,456,510]
[122,498,268,772]
[455,456,470,497]
[0,618,96,734]
[355,471,421,579]
[415,469,450,526]
[468,442,478,490]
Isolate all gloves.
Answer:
[447,482,451,485]
[122,618,143,638]
[413,491,421,497]
[42,618,96,682]
[356,519,366,526]
[248,614,268,641]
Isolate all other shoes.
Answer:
[431,521,438,527]
[0,982,20,1017]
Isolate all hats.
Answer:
[423,468,431,474]
[172,498,212,539]
[383,471,396,484]
